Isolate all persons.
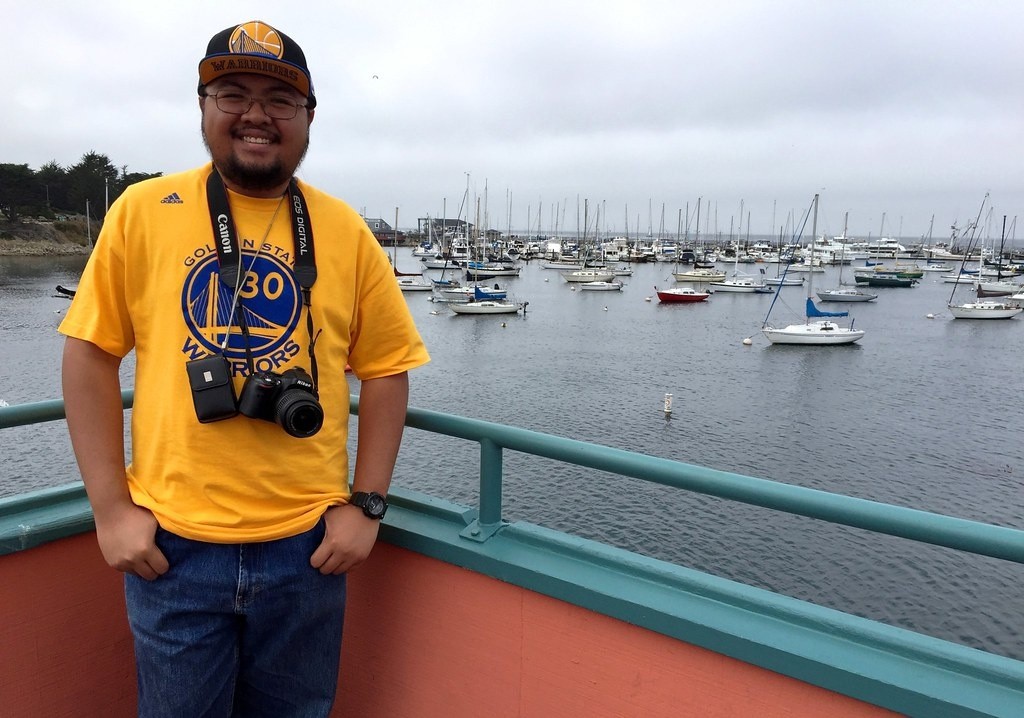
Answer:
[55,18,433,717]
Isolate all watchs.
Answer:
[348,492,389,518]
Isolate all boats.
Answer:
[755,287,776,294]
[654,285,710,302]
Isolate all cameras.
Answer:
[237,365,323,439]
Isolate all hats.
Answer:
[197,20,315,97]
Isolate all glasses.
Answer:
[202,89,305,119]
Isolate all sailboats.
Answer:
[709,198,767,293]
[579,200,624,291]
[762,226,807,286]
[760,193,868,346]
[448,196,529,314]
[946,189,1024,319]
[816,211,878,302]
[56,178,109,297]
[393,207,433,291]
[412,171,1024,306]
